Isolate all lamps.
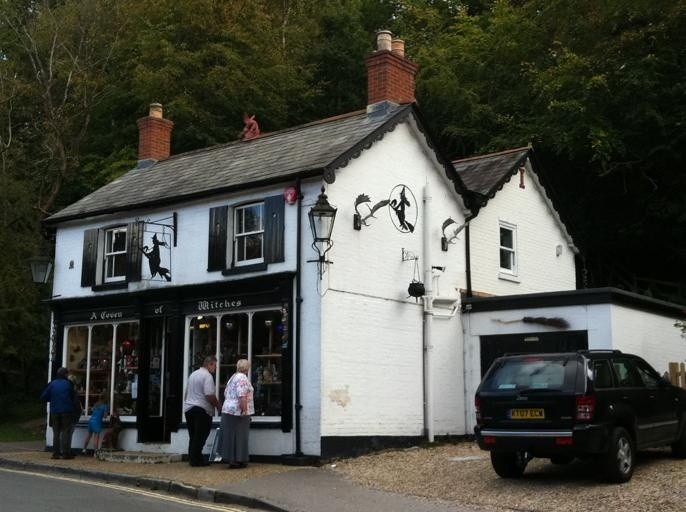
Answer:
[28,255,54,284]
[304,184,337,279]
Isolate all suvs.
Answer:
[474,350,686,483]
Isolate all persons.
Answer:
[217,359,257,469]
[180,357,224,468]
[39,367,81,460]
[80,391,109,455]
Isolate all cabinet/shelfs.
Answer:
[72,355,138,416]
[219,352,284,416]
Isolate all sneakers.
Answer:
[230,460,248,468]
[80,447,100,458]
